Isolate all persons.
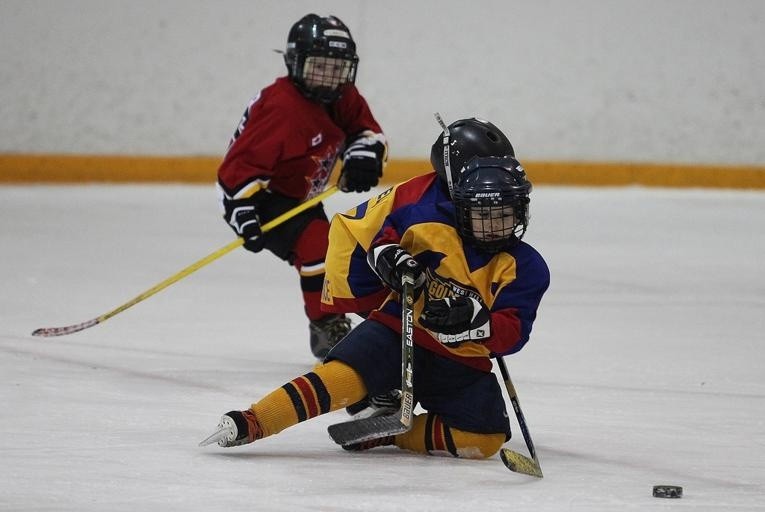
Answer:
[320,117,515,413]
[216,12,389,360]
[216,154,551,461]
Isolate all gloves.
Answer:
[222,198,265,253]
[338,142,382,194]
[417,293,494,348]
[364,242,428,303]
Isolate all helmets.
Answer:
[284,11,359,105]
[430,117,531,249]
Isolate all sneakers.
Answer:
[368,386,403,416]
[308,311,353,359]
[218,410,259,449]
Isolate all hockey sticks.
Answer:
[327,274,413,447]
[497,355,542,478]
[32,183,339,337]
